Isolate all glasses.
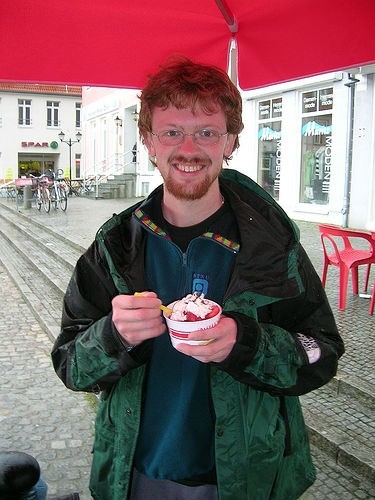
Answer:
[148,130,230,146]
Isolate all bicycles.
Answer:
[29,169,53,213]
[45,170,69,211]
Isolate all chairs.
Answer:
[64,178,80,197]
[318,224,375,316]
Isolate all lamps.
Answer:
[114,116,122,127]
[131,109,138,121]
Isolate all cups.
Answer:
[164,299,222,350]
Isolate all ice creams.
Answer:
[168,290,220,321]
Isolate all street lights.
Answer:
[58,130,83,195]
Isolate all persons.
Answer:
[51,58,345,500]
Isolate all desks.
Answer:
[69,178,84,189]
